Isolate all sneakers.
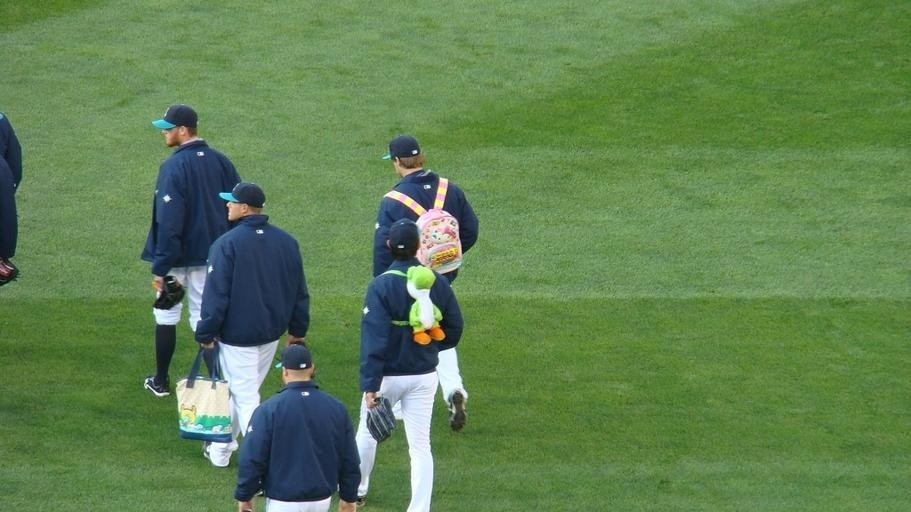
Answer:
[447,387,467,431]
[142,376,171,398]
[355,494,367,508]
[203,445,214,460]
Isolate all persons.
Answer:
[373,135,480,429]
[141,104,242,397]
[236,345,364,512]
[347,217,467,510]
[196,183,311,469]
[1,112,23,287]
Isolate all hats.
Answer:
[275,344,312,371]
[381,135,420,160]
[387,218,419,255]
[218,182,267,211]
[151,104,199,130]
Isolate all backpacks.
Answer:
[383,175,465,277]
[384,265,445,346]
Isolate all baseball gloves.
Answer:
[366,399,396,444]
[154,275,183,310]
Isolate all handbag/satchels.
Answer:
[175,340,234,444]
